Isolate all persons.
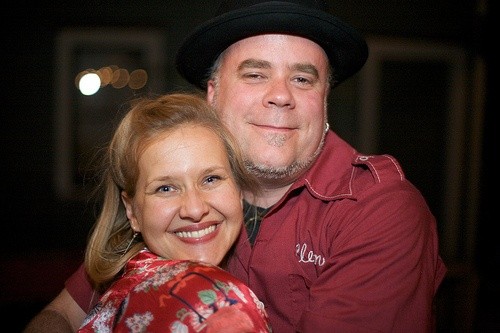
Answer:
[76,95,273,333]
[25,1,448,333]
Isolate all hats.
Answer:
[175,0,369,91]
